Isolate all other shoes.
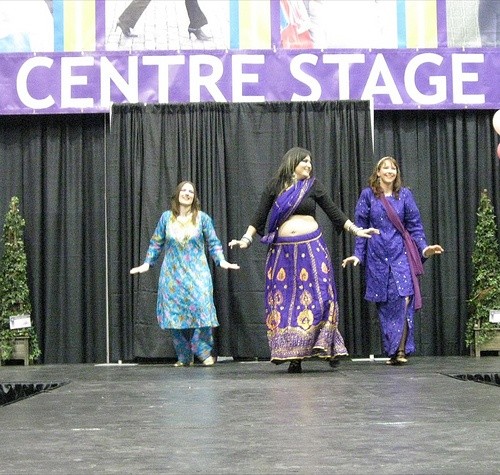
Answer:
[172,360,193,367]
[201,355,215,366]
[385,351,408,366]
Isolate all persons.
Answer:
[114,0,215,41]
[130,180,240,367]
[228,147,380,373]
[342,157,444,365]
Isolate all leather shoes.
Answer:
[329,355,353,368]
[287,360,302,373]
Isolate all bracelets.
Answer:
[240,238,250,246]
[242,234,254,243]
[347,223,363,237]
